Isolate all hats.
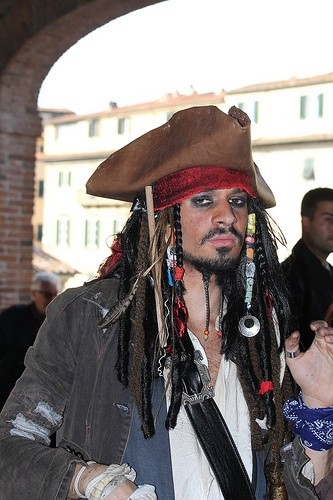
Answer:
[87,104,276,208]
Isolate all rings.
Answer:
[286,347,301,357]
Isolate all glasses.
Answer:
[35,290,57,298]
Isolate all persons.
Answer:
[0,271,58,414]
[0,106,333,500]
[281,188,333,353]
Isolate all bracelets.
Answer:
[74,461,96,498]
